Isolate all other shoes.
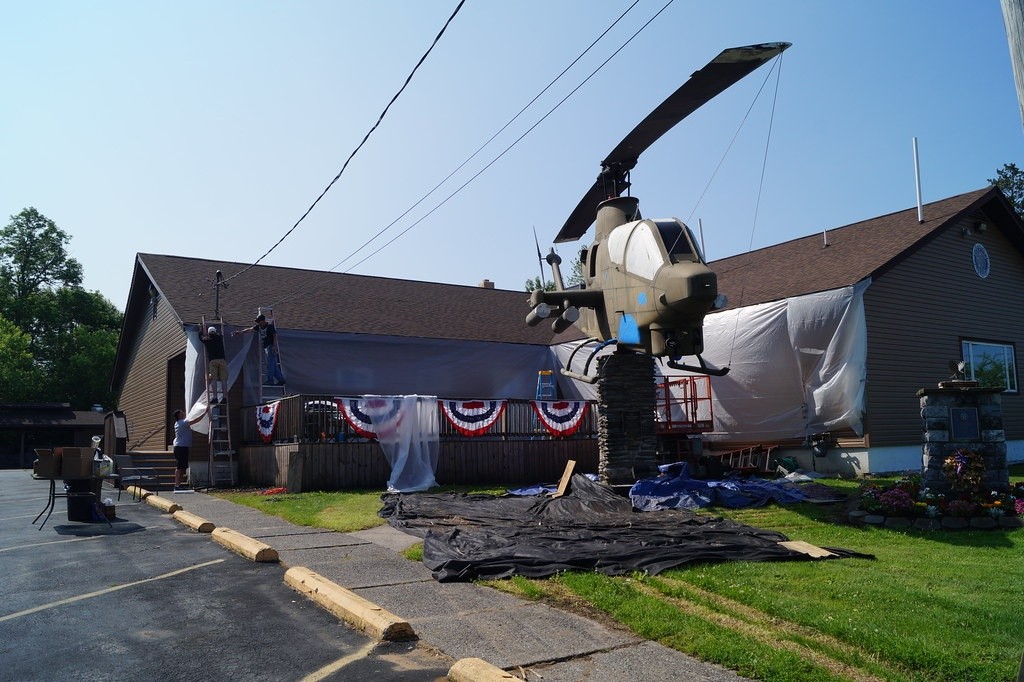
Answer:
[210,398,218,403]
[274,378,286,386]
[174,485,188,490]
[219,398,227,404]
[263,380,274,385]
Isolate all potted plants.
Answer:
[976,352,1008,391]
[937,359,977,388]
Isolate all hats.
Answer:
[255,315,266,322]
[207,326,217,334]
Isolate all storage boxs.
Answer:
[33,446,113,476]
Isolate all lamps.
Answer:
[147,282,157,305]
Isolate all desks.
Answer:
[32,475,119,531]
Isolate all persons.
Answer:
[231,314,286,386]
[173,407,208,490]
[198,323,228,403]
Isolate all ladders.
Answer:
[712,443,778,473]
[258,307,288,404]
[536,370,557,399]
[202,316,235,486]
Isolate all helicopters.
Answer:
[525,40,793,384]
